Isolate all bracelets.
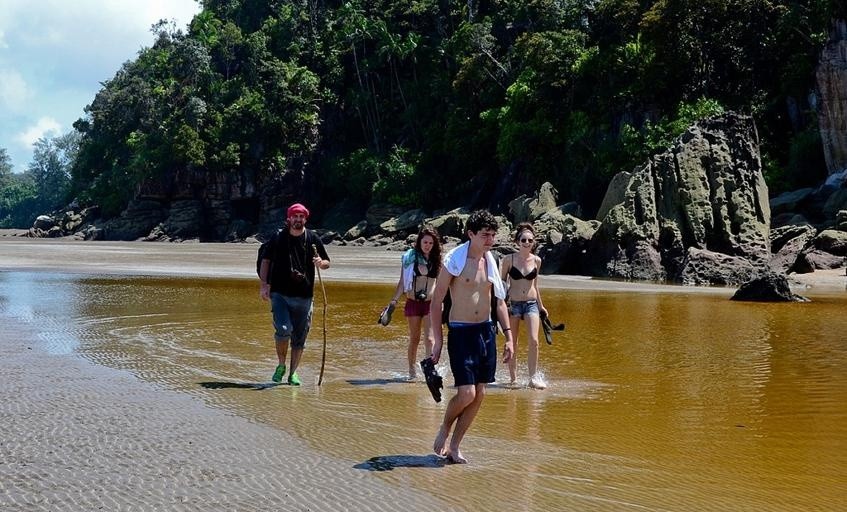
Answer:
[503,328,511,333]
[392,299,397,303]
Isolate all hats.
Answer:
[286,202,310,218]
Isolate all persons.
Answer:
[260,204,331,386]
[430,209,515,463]
[501,222,548,388]
[390,228,442,383]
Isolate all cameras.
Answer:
[415,289,427,300]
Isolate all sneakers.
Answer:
[526,373,547,389]
[378,303,395,327]
[419,358,443,404]
[509,376,520,389]
[271,362,287,383]
[287,371,301,386]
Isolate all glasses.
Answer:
[519,238,533,244]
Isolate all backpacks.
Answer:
[255,229,284,281]
